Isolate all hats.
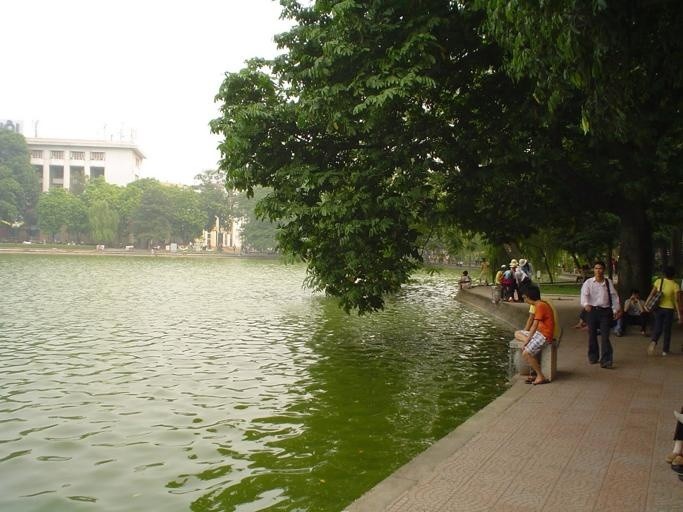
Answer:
[509,259,527,267]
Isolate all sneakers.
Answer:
[616,332,622,336]
[665,452,683,465]
[590,359,611,367]
[674,411,683,423]
[640,331,648,337]
[648,341,671,355]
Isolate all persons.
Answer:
[665,405,682,473]
[455,258,682,386]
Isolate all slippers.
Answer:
[525,375,549,384]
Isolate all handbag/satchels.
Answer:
[646,292,663,311]
[609,308,617,328]
